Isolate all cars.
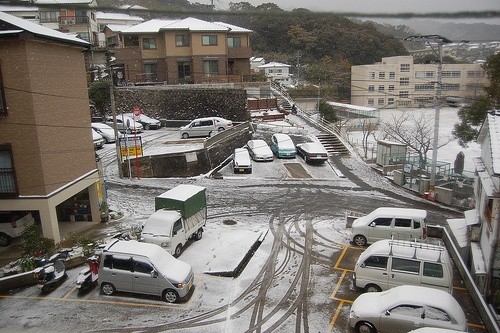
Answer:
[269,133,296,160]
[348,284,467,333]
[179,117,233,139]
[90,122,122,144]
[92,127,106,150]
[295,142,329,163]
[244,140,274,162]
[117,113,162,131]
[104,115,144,134]
[232,148,252,174]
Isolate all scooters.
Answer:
[74,251,100,294]
[34,248,74,295]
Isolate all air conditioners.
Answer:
[61,20,76,24]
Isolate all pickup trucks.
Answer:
[0,212,37,247]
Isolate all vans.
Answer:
[349,207,429,246]
[353,233,454,294]
[97,237,194,304]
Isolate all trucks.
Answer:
[140,184,208,258]
